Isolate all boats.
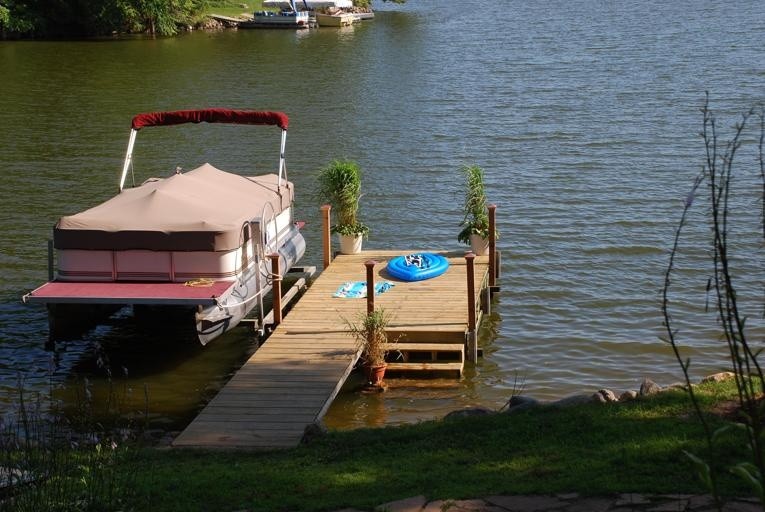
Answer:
[316,14,353,27]
[21,108,307,348]
[238,10,310,29]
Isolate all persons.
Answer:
[414,253,423,267]
[404,254,416,265]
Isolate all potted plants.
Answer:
[335,303,408,384]
[458,157,503,256]
[310,159,371,256]
